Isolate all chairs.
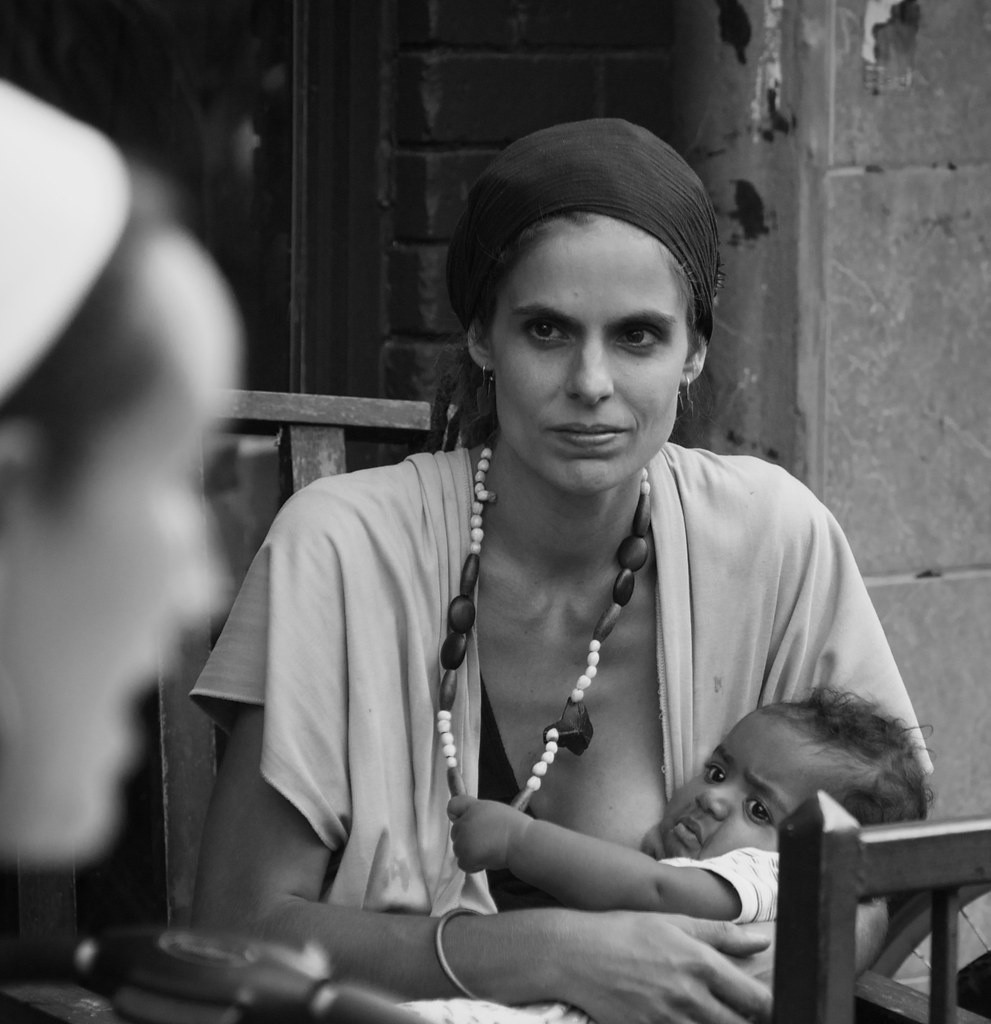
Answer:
[0,391,471,1024]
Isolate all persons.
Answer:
[400,687,932,1024]
[0,78,246,875]
[189,121,932,1024]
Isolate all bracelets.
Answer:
[435,907,490,1001]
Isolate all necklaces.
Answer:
[438,436,651,813]
